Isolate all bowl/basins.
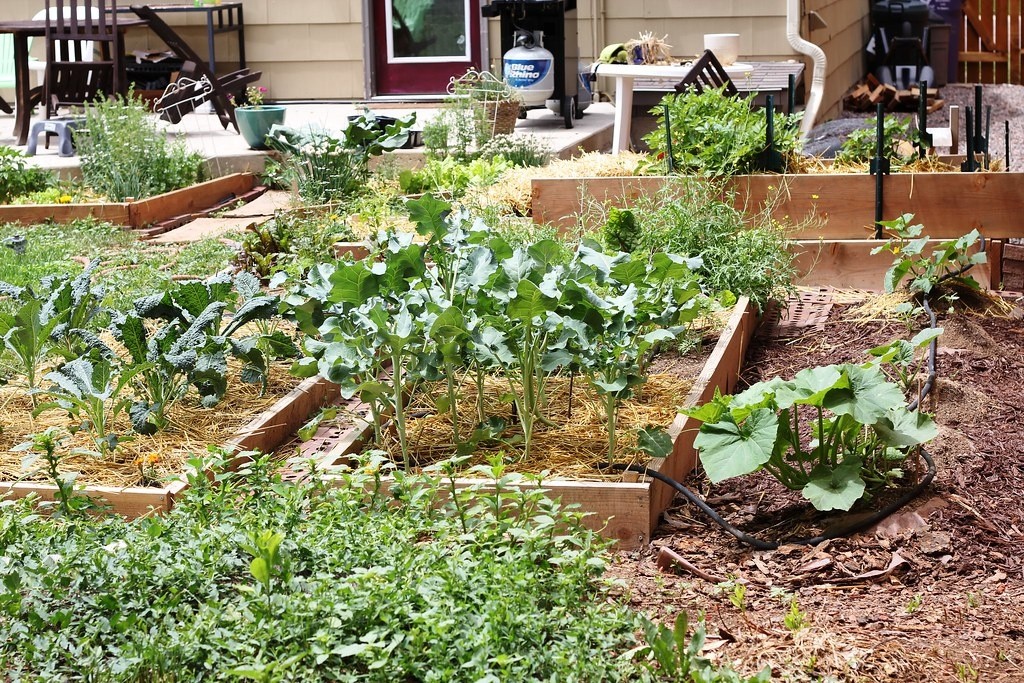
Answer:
[704,34,740,64]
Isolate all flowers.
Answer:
[227,86,268,110]
[459,47,527,111]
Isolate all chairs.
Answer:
[874,37,935,89]
[43,0,127,149]
[0,33,33,89]
[14,6,99,118]
[675,49,744,107]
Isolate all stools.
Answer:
[24,117,102,158]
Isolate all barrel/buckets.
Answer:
[869,0,926,90]
[924,0,958,82]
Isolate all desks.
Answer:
[0,18,150,136]
[101,2,247,106]
[595,61,755,157]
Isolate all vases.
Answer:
[234,105,287,151]
[703,33,741,66]
